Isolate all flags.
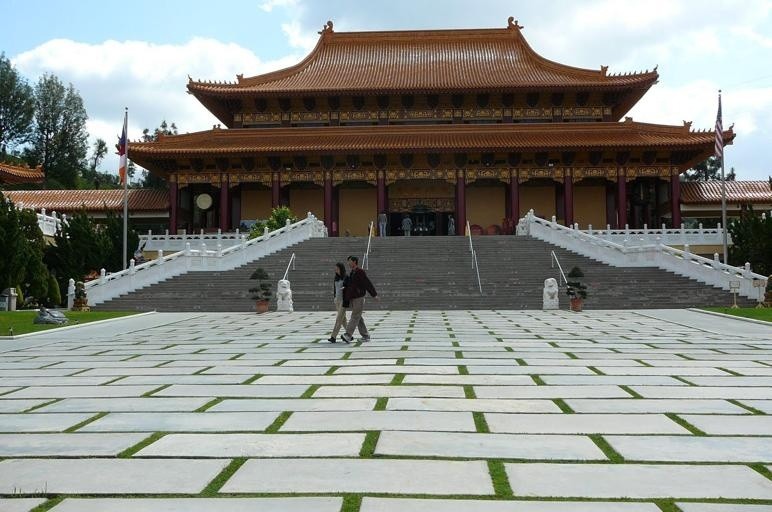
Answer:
[714,107,723,158]
[119,124,125,183]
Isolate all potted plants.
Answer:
[566,267,587,312]
[248,268,273,313]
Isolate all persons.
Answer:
[341,256,380,344]
[448,215,455,235]
[328,262,353,343]
[401,214,413,236]
[378,211,387,238]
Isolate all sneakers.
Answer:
[328,337,336,343]
[341,334,354,344]
[357,335,370,342]
